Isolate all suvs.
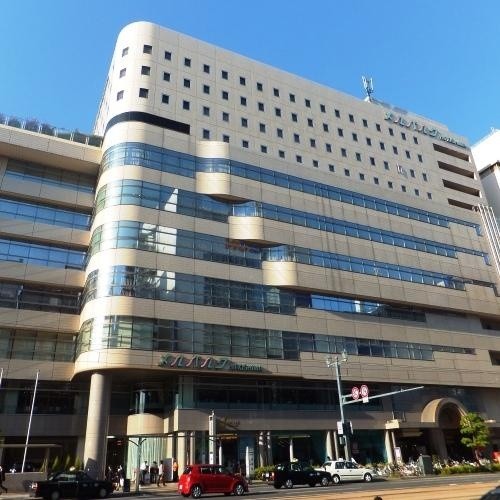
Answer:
[272,463,331,488]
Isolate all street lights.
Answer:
[326,349,354,459]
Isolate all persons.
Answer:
[140,460,167,488]
[173,459,178,482]
[0,465,8,495]
[105,463,125,491]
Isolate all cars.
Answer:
[315,459,376,484]
[178,464,248,498]
[30,471,115,500]
